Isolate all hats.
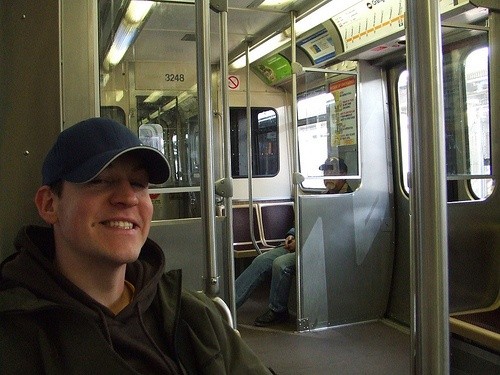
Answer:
[40,117,173,186]
[318,156,348,173]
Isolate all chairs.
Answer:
[218,202,294,258]
[448,291,500,352]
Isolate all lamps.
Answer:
[101,0,159,72]
[227,0,363,71]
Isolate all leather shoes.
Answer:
[255,308,291,327]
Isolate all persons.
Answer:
[0,117,275,375]
[235,157,353,326]
